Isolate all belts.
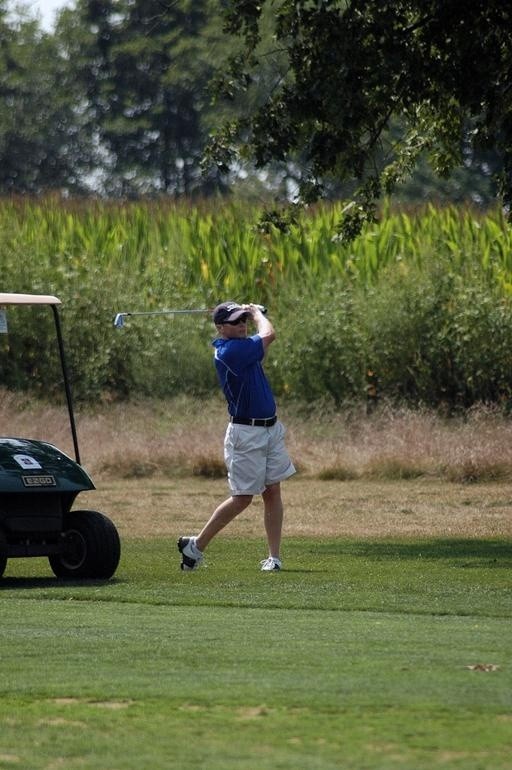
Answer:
[233,416,277,426]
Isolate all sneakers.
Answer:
[177,536,202,570]
[261,559,282,571]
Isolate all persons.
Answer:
[176,300,298,572]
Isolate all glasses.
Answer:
[218,315,246,325]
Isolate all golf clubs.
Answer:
[113,306,268,328]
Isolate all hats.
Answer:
[213,302,252,322]
